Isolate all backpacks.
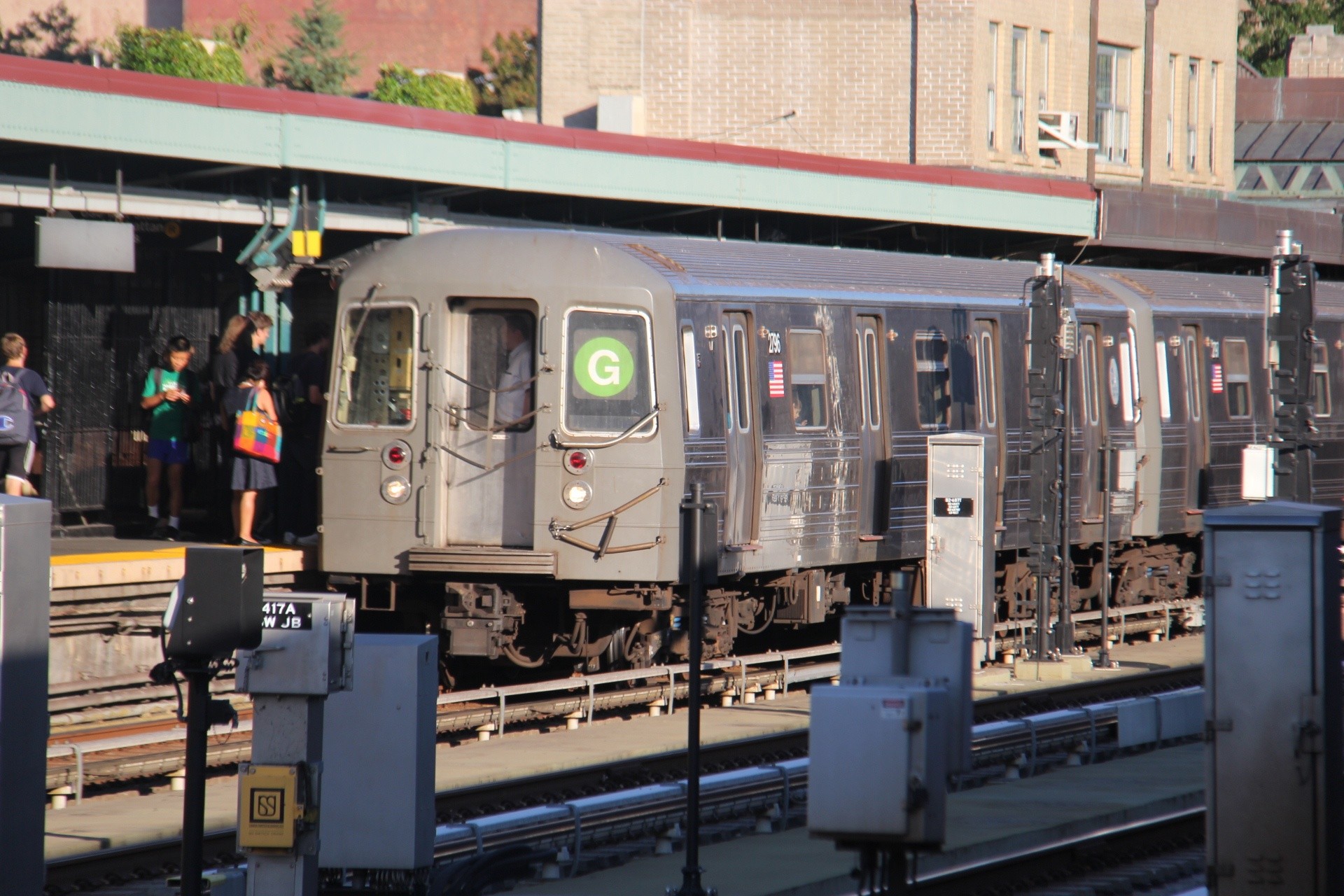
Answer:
[0,368,32,445]
[154,365,203,442]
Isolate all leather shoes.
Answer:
[237,536,268,547]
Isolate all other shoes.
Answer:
[144,515,160,534]
[163,526,178,542]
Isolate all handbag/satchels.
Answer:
[233,384,283,465]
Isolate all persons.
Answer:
[140,336,205,542]
[489,320,531,431]
[207,311,332,546]
[1,332,56,496]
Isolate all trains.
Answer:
[285,186,1344,674]
